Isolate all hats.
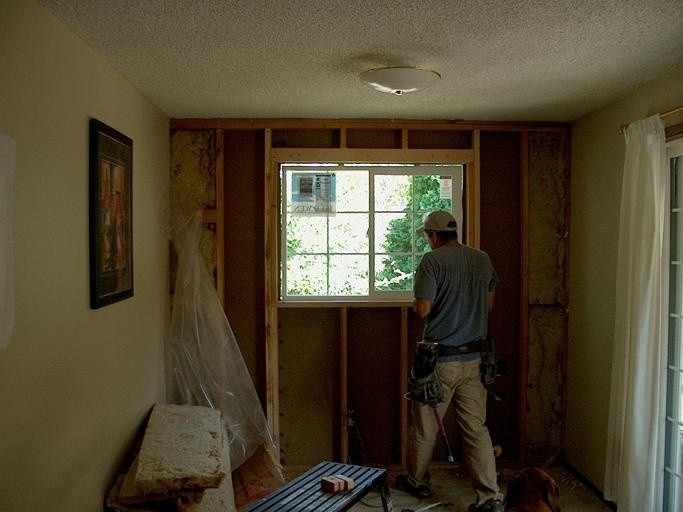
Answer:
[413,210,457,235]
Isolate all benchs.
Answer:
[244,461,388,512]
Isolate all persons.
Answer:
[395,210,506,512]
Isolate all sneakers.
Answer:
[467,499,501,512]
[393,471,433,500]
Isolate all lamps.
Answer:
[358,57,441,95]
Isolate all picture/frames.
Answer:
[87,116,134,310]
